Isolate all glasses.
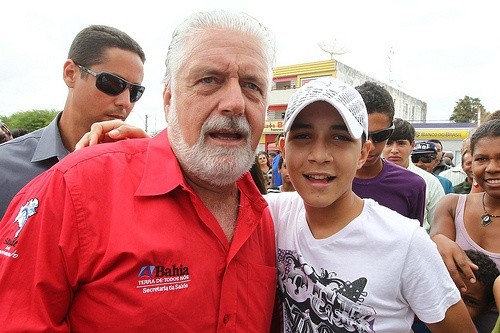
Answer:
[368,122,395,143]
[411,155,436,163]
[76,63,145,102]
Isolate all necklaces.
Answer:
[479,193,499,227]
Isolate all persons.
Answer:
[1,12,278,333]
[74,75,480,333]
[252,81,500,333]
[0,25,147,220]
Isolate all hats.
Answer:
[283,76,368,142]
[412,142,437,154]
